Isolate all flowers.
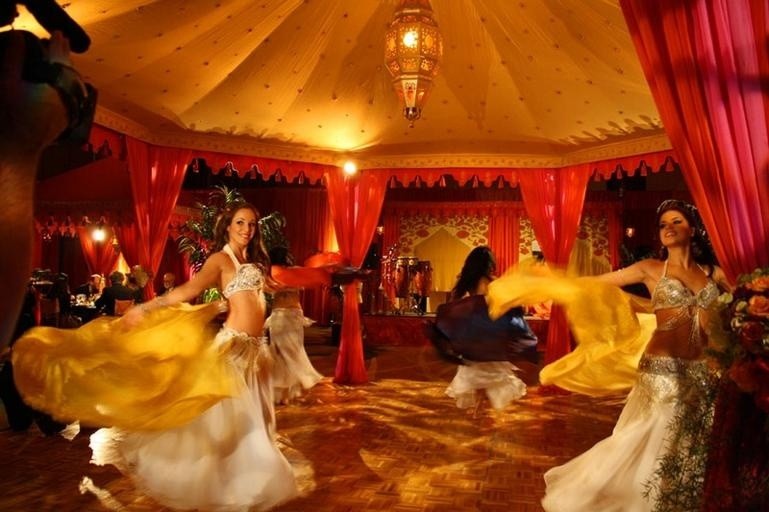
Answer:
[702,265,767,371]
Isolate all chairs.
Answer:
[29,281,136,328]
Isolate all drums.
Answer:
[396,256,432,297]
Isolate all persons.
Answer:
[45,272,176,325]
[485,199,734,512]
[0,30,89,362]
[11,202,298,512]
[264,247,324,406]
[421,246,537,420]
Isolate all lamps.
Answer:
[381,0,444,130]
